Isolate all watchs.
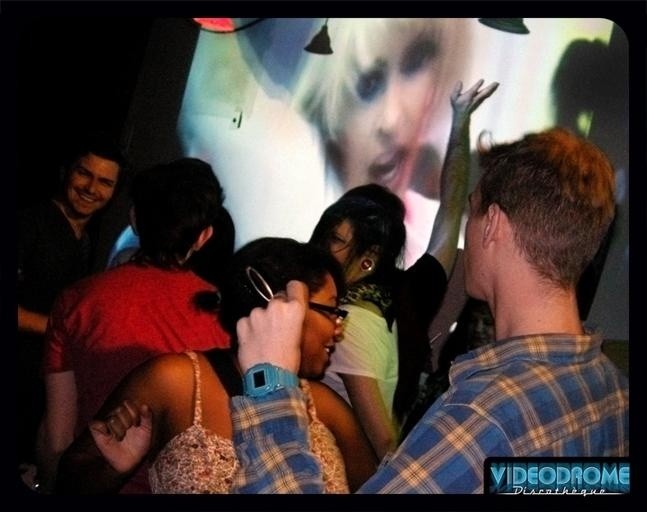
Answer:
[237,362,302,398]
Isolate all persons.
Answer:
[228,124,628,494]
[342,79,501,438]
[2,129,400,497]
[290,18,466,225]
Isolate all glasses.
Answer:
[307,300,348,326]
